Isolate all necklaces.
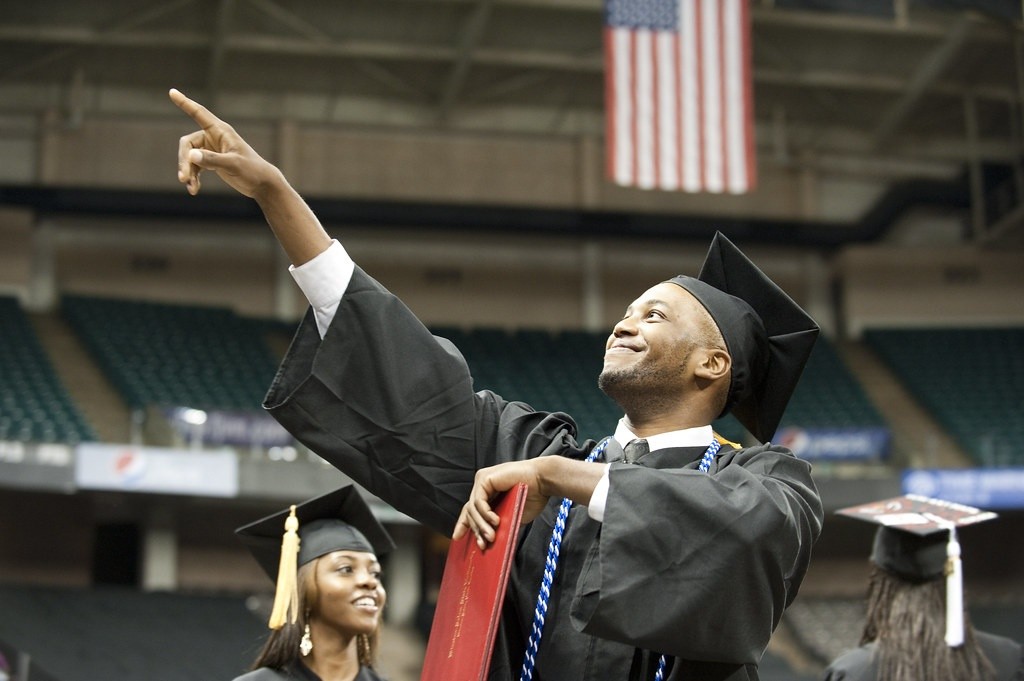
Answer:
[518,435,722,681]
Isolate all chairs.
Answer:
[898,324,1024,465]
[426,323,894,461]
[0,289,304,451]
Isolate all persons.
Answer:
[821,493,1024,680]
[229,482,398,681]
[165,86,827,681]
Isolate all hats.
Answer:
[236,484,396,629]
[835,493,998,647]
[661,231,821,444]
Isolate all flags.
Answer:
[604,0,758,196]
